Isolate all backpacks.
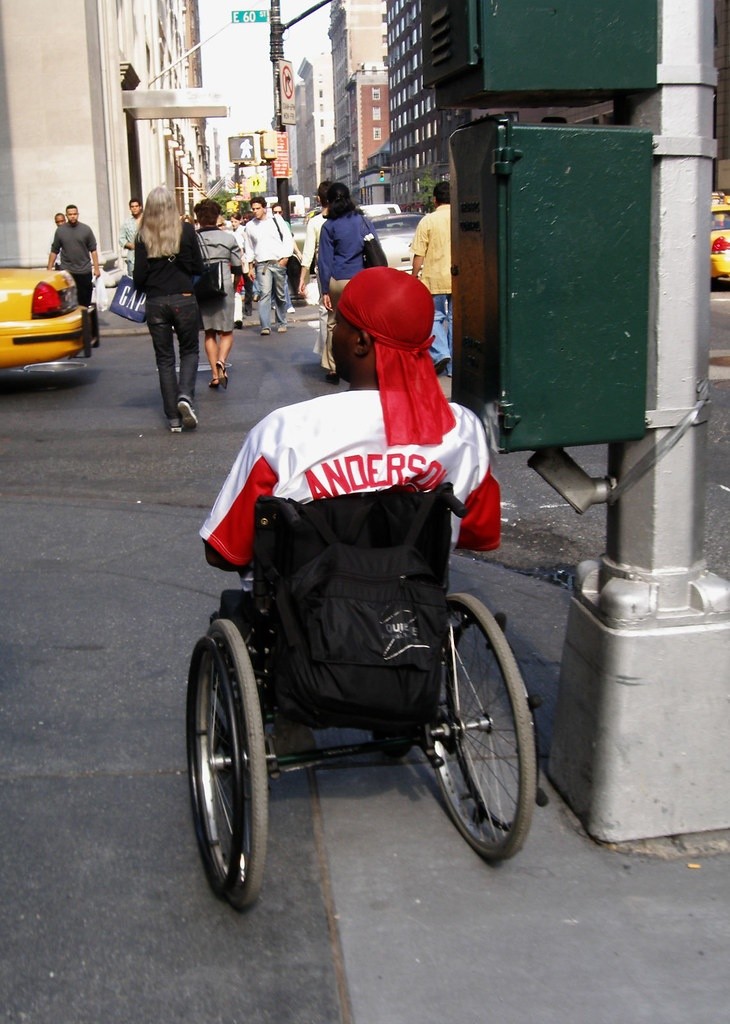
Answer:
[273,490,450,727]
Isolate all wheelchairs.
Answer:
[183,482,550,912]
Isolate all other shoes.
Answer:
[234,320,243,329]
[434,358,451,375]
[286,306,296,314]
[245,310,251,316]
[325,373,339,385]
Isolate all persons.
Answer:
[181,203,295,329]
[298,180,334,360]
[119,198,144,280]
[319,182,382,385]
[47,205,100,309]
[133,187,204,432]
[409,181,453,378]
[55,213,66,270]
[194,198,243,392]
[244,196,295,334]
[199,267,502,756]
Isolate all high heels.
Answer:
[216,360,228,390]
[208,379,220,391]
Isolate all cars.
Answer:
[263,195,429,276]
[0,263,102,372]
[709,192,730,285]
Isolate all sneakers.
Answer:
[277,324,287,333]
[168,421,183,433]
[260,328,272,335]
[176,395,198,429]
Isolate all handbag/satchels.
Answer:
[90,275,109,312]
[193,235,228,312]
[108,275,146,324]
[287,254,303,279]
[362,232,388,269]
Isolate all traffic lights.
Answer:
[379,171,384,182]
[228,134,258,163]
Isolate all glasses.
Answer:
[217,223,223,227]
[274,210,283,214]
